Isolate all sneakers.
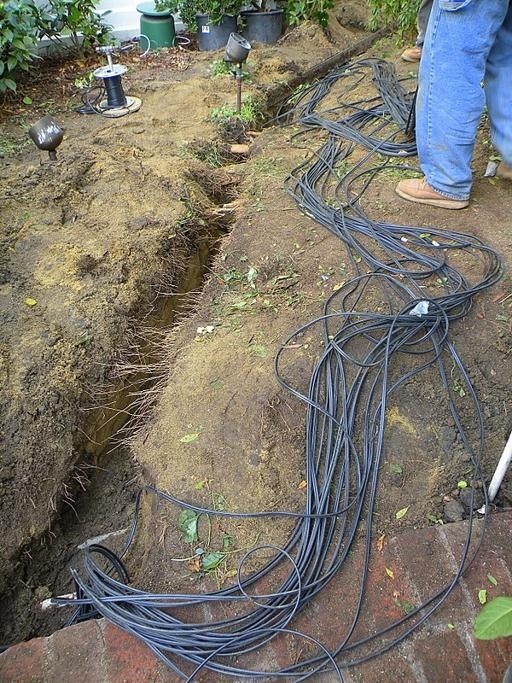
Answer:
[394,175,471,213]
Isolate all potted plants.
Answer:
[153,0,311,51]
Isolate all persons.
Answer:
[398,0,434,63]
[393,0,512,213]
